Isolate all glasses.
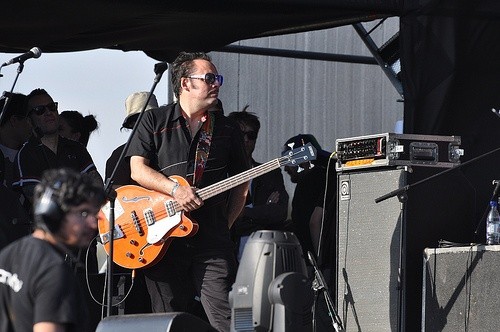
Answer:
[241,130,257,140]
[188,73,223,86]
[28,102,58,117]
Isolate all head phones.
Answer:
[32,174,70,233]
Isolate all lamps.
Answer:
[229,230,314,332]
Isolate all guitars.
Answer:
[97,142,318,270]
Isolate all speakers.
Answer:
[95,311,218,332]
[421,244,500,332]
[336,166,475,332]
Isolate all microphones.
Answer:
[48,227,84,269]
[1,46,42,67]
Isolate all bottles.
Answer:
[486,200,500,245]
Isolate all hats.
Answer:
[119,91,159,130]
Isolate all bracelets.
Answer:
[170,182,179,197]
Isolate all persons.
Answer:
[229,110,289,261]
[11,89,102,274]
[130,52,244,332]
[0,164,104,332]
[0,93,32,251]
[284,133,338,273]
[105,92,158,187]
[58,110,99,149]
